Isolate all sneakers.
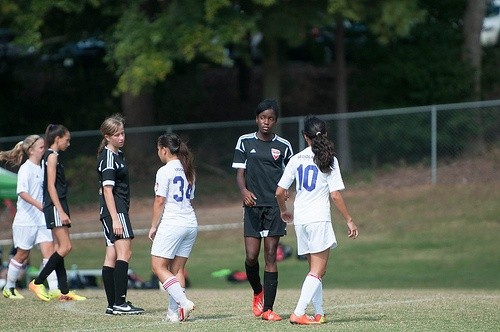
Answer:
[261,309,282,321]
[28,279,51,301]
[112,301,145,314]
[289,312,317,324]
[48,289,59,299]
[252,289,264,317]
[2,287,24,299]
[105,305,112,315]
[162,314,179,323]
[315,314,327,323]
[176,300,196,321]
[58,291,87,302]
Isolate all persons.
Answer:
[275,116,359,324]
[148,133,198,322]
[97,118,145,315]
[28,124,87,302]
[232,99,294,321]
[0,135,61,299]
